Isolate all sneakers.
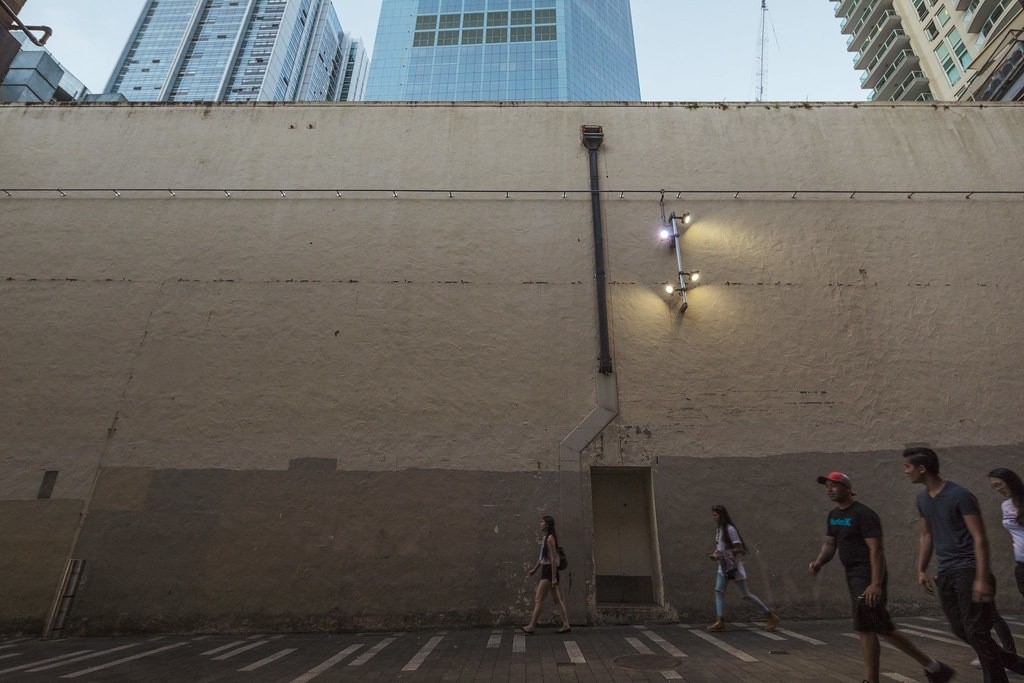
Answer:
[706,619,726,631]
[764,613,780,631]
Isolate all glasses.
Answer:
[992,481,1006,491]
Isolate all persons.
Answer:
[707,505,781,631]
[901,447,1024,683]
[519,516,573,634]
[808,471,957,683]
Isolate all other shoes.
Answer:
[923,660,955,683]
[519,626,534,634]
[553,627,572,634]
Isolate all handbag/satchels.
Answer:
[732,555,747,582]
[556,546,567,570]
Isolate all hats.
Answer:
[816,471,852,489]
